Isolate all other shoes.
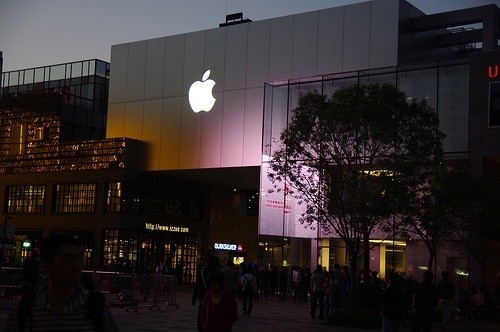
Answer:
[243,310,246,316]
[247,312,250,317]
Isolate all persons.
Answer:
[436,270,455,327]
[382,274,406,332]
[22,251,40,294]
[409,271,436,332]
[190,254,239,332]
[236,262,311,315]
[3,233,119,332]
[310,264,378,320]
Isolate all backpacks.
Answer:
[243,275,257,298]
